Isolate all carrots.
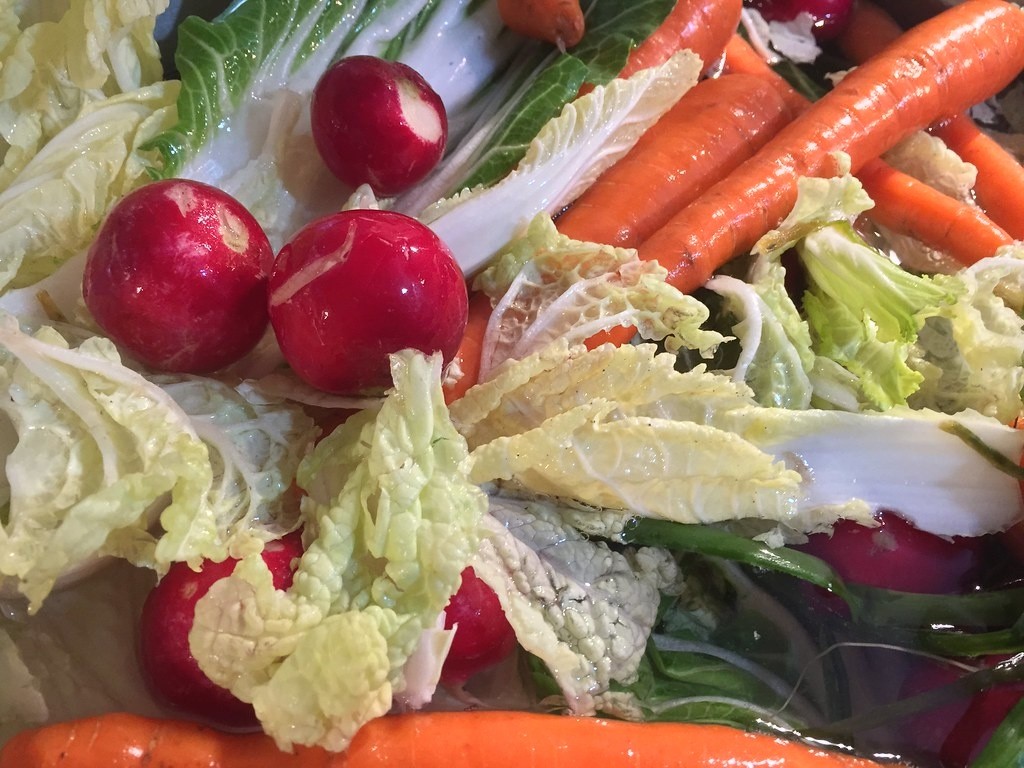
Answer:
[837,2,1022,245]
[496,0,586,44]
[0,712,913,768]
[704,35,1015,270]
[592,0,1024,352]
[444,69,797,403]
[586,1,746,98]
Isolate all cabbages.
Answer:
[0,0,1023,768]
